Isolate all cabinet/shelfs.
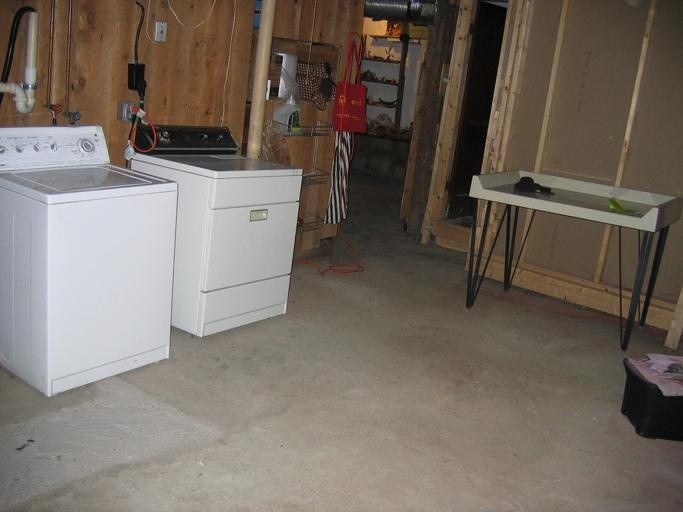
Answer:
[261,37,341,233]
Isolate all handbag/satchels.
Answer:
[332,81,367,134]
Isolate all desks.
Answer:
[466,170,682,351]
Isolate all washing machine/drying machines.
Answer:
[132,128,306,338]
[1,124,180,398]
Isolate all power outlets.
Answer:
[117,101,139,121]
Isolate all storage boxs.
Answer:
[621,358,683,441]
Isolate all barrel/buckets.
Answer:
[272,93,302,135]
